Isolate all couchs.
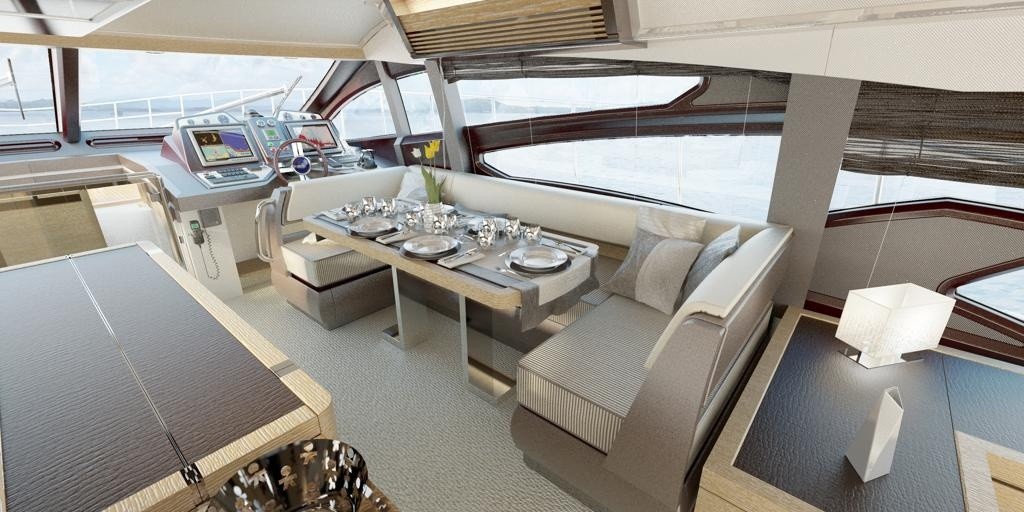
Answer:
[263,164,796,512]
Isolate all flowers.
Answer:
[411,139,450,204]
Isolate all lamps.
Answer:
[833,284,957,368]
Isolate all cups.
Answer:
[477,216,543,250]
[405,201,460,236]
[341,193,400,223]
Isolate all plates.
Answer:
[400,236,461,261]
[402,235,458,256]
[348,225,400,239]
[504,247,573,278]
[349,218,392,235]
[510,246,568,272]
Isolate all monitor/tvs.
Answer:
[187,124,259,168]
[284,120,343,155]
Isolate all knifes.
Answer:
[380,231,407,240]
[441,247,477,264]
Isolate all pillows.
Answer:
[396,172,447,202]
[599,221,706,315]
[637,205,706,243]
[681,224,741,304]
